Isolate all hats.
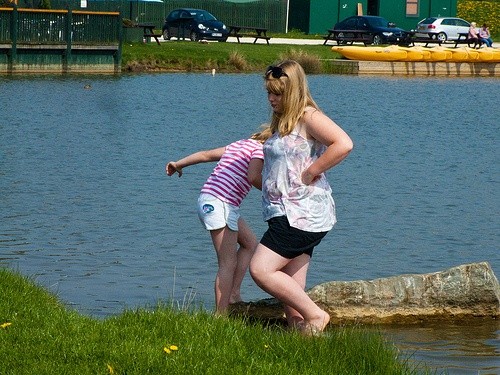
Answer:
[482,24,488,28]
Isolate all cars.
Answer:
[162,8,231,43]
[333,16,416,46]
[414,16,482,44]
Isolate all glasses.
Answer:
[266,66,288,78]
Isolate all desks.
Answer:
[322,30,368,47]
[454,33,485,49]
[131,24,161,46]
[397,30,442,47]
[220,24,270,46]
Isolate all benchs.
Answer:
[396,35,444,43]
[144,33,163,37]
[222,32,272,40]
[321,36,372,41]
[452,38,485,44]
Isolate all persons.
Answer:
[166,123,275,318]
[467,21,481,50]
[478,23,493,47]
[248,62,353,335]
